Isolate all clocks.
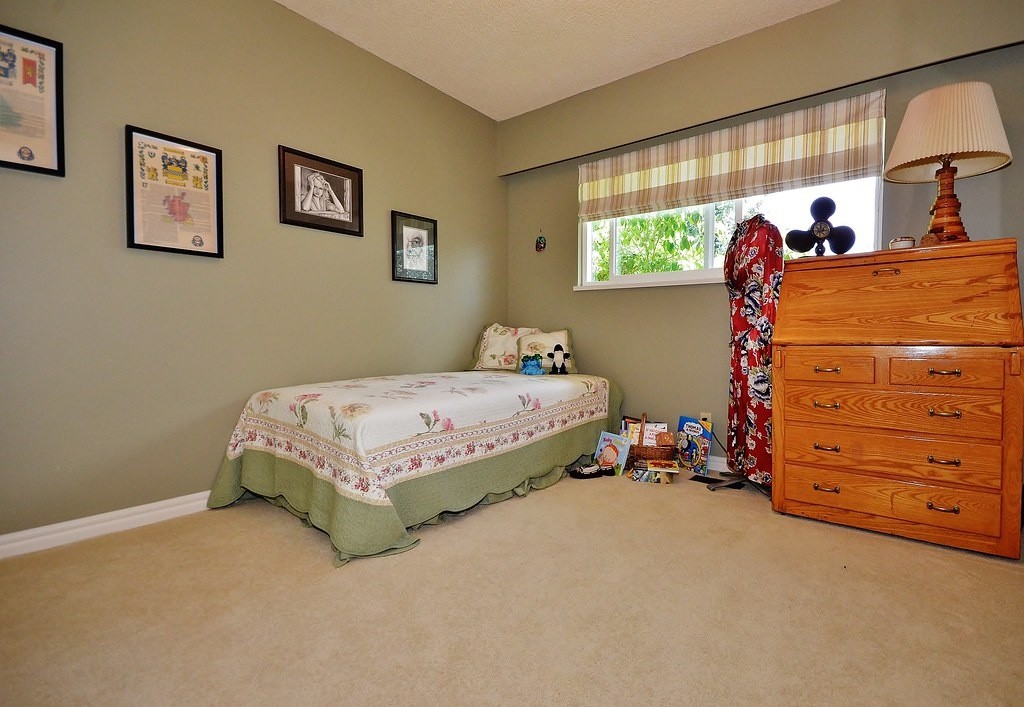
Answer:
[786,197,856,259]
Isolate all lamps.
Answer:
[883,82,1012,246]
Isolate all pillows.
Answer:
[517,328,579,374]
[465,322,544,374]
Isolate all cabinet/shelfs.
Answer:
[771,238,1024,560]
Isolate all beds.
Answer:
[207,371,624,569]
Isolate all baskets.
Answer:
[629,412,677,460]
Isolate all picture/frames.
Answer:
[391,210,438,284]
[278,145,364,238]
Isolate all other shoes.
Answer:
[570,460,615,479]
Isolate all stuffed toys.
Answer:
[547,344,571,375]
